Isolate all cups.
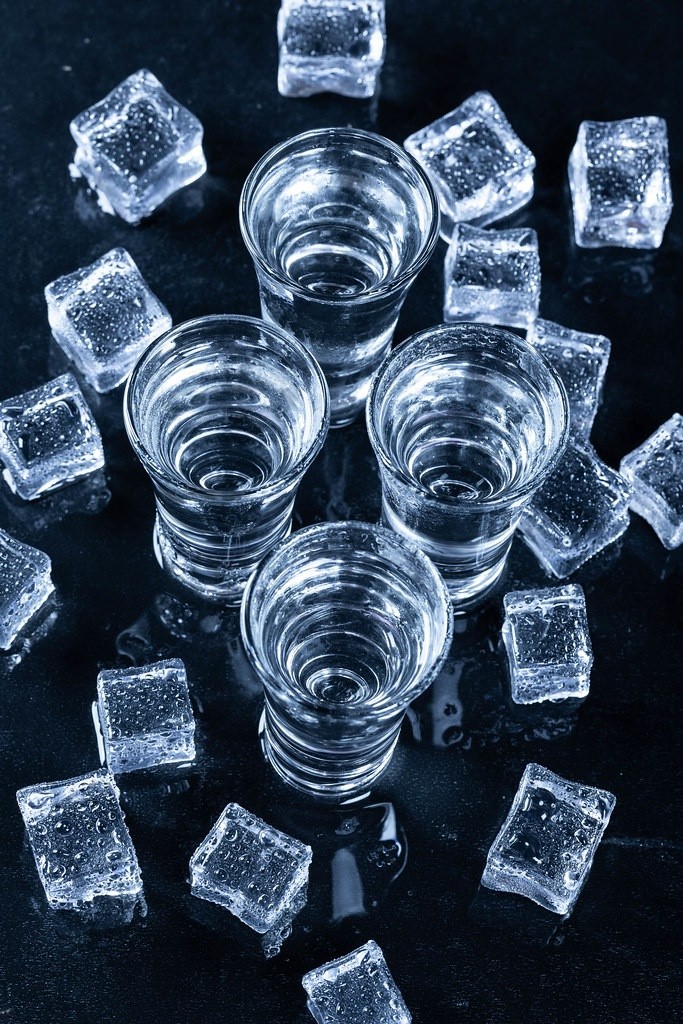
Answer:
[150,510,300,600]
[123,314,330,545]
[318,342,393,434]
[241,523,453,742]
[241,125,442,365]
[380,491,514,608]
[365,319,570,555]
[252,699,400,800]
